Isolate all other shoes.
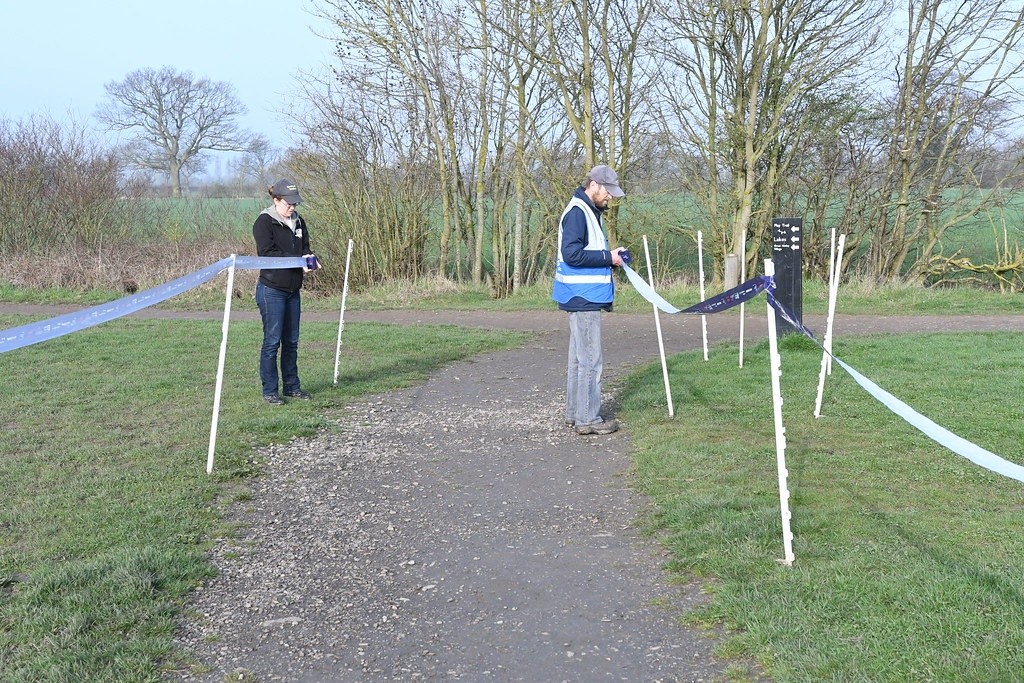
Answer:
[263,389,312,405]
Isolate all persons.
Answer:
[551,165,629,435]
[252,179,312,406]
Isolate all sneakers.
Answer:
[567,417,619,435]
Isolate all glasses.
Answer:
[277,198,300,208]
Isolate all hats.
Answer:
[587,165,626,198]
[272,178,304,204]
